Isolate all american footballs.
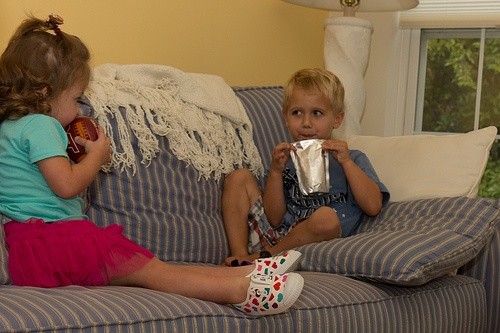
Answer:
[66,115,99,163]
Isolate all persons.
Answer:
[0,12,305,316]
[222,67,390,266]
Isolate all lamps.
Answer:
[284,0,420,136]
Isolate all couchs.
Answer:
[0,84,500,333]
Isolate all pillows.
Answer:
[347,125,497,203]
[273,196,500,288]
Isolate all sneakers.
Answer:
[231,271,305,317]
[244,249,302,278]
